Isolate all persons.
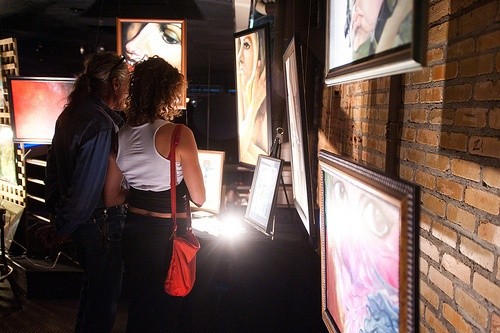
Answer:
[44,51,131,333]
[103,57,205,333]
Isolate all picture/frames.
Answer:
[244,155,286,236]
[323,0,428,88]
[7,76,77,146]
[115,17,187,110]
[317,148,419,333]
[198,150,226,216]
[282,34,318,250]
[231,21,274,170]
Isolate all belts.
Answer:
[85,204,128,223]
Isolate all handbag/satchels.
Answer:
[165,232,200,296]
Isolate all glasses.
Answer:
[109,54,127,78]
